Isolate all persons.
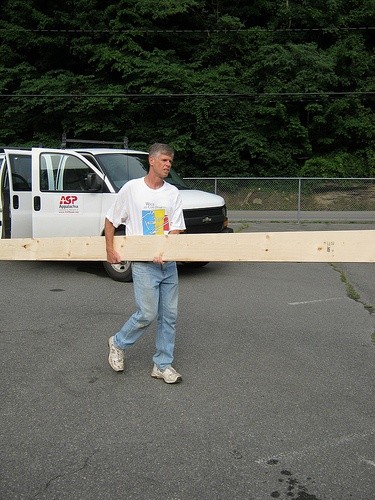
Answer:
[104,143,185,383]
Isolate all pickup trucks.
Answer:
[1,147,234,284]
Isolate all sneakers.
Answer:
[108,335,125,372]
[151,364,182,384]
